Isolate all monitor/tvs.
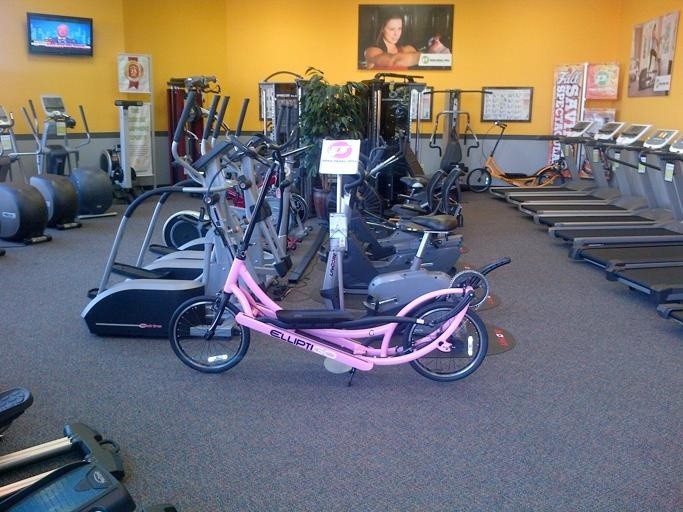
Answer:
[26,12,93,56]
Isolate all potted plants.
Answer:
[289,64,372,218]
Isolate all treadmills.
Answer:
[488,119,683,324]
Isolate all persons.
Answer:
[647,23,662,73]
[363,13,450,68]
[49,24,77,44]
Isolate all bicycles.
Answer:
[168,125,490,389]
[468,120,567,193]
[322,132,461,233]
